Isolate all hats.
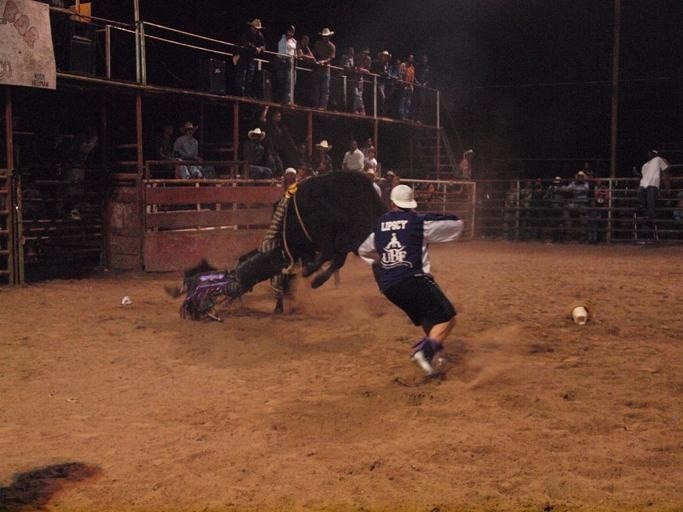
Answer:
[286,167,296,175]
[179,121,198,133]
[248,17,265,31]
[390,184,418,209]
[315,140,332,151]
[575,171,587,179]
[247,127,266,140]
[317,27,334,38]
[465,149,475,157]
[378,49,392,60]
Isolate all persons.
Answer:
[144,120,218,187]
[239,103,333,180]
[269,25,430,124]
[341,138,395,209]
[356,184,467,375]
[453,144,683,248]
[231,19,266,97]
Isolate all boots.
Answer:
[414,339,443,374]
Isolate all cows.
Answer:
[273,169,389,314]
